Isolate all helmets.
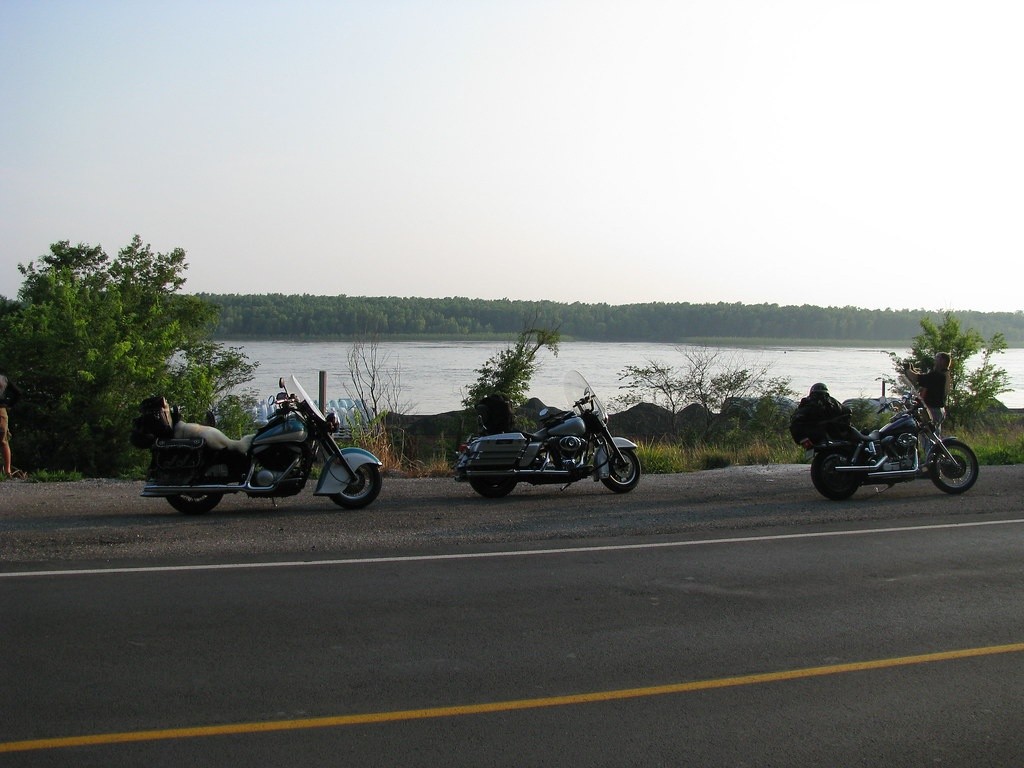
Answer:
[810,383,828,403]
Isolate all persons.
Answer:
[0,375,11,477]
[902,351,949,454]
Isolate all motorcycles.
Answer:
[136,374,384,516]
[451,369,642,499]
[799,374,980,501]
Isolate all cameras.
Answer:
[903,364,913,370]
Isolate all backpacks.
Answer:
[140,395,173,435]
